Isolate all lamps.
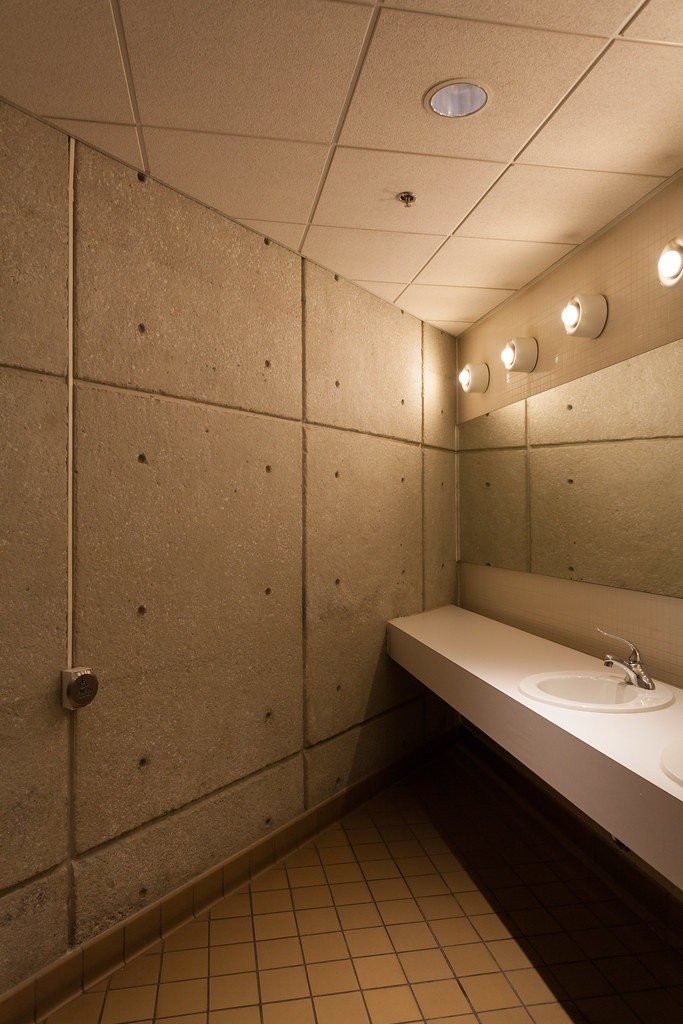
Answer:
[658,236,683,289]
[501,339,538,373]
[422,76,492,122]
[562,293,608,339]
[459,362,489,393]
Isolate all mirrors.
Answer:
[455,338,683,598]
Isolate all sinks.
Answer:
[518,669,676,714]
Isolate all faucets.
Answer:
[596,626,656,691]
